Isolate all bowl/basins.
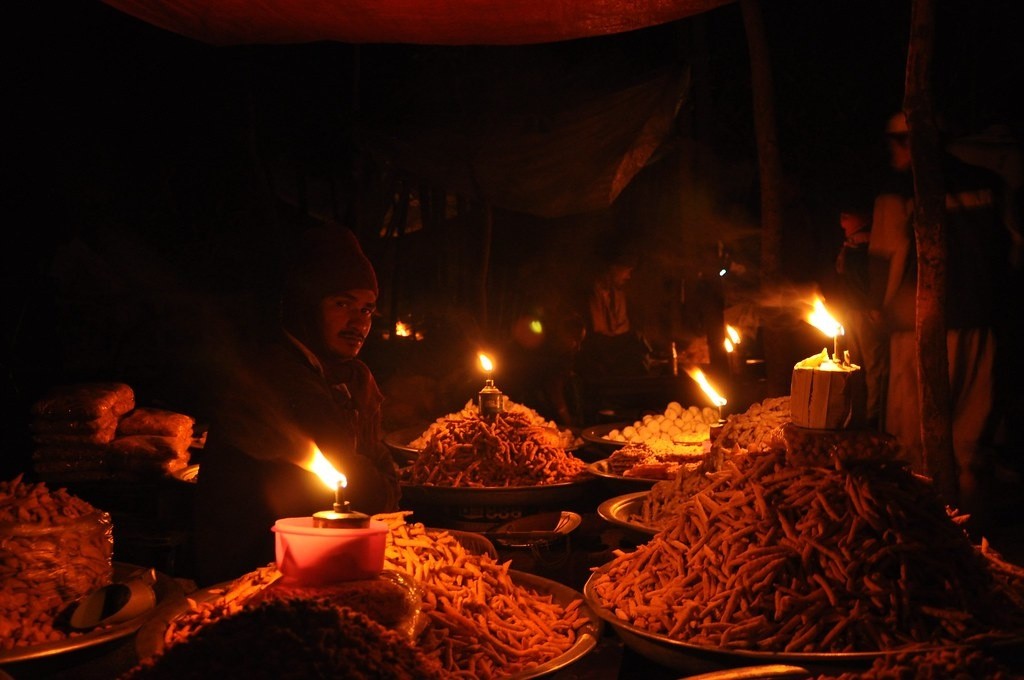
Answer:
[0,420,1024,680]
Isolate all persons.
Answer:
[543,289,675,429]
[193,223,404,582]
[835,107,1024,506]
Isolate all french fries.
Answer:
[398,412,591,486]
[585,429,1023,680]
[161,509,592,680]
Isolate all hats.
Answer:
[286,222,380,307]
